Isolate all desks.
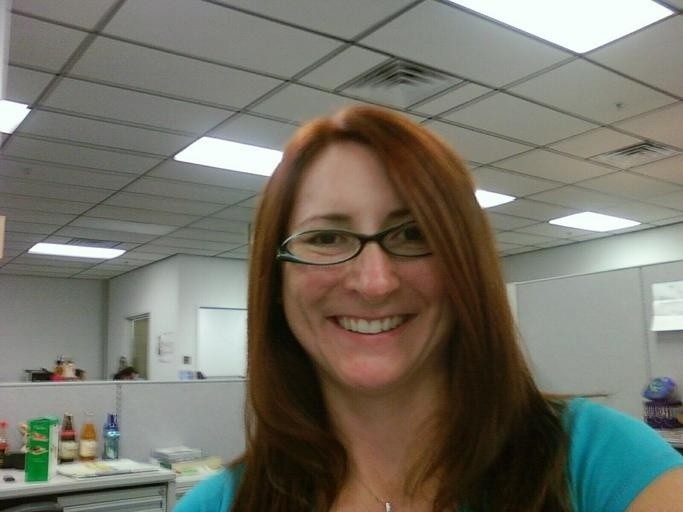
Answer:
[1,456,177,512]
[656,431,683,451]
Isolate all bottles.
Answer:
[102,413,120,459]
[0,421,9,466]
[78,412,97,461]
[58,414,76,462]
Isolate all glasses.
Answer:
[275,218,434,266]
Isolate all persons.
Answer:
[113,366,140,381]
[165,103,683,512]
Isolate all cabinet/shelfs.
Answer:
[170,466,226,512]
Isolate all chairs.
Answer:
[5,502,65,512]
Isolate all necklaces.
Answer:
[346,466,416,511]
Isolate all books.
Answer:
[642,400,682,447]
[149,440,227,505]
[56,456,161,479]
